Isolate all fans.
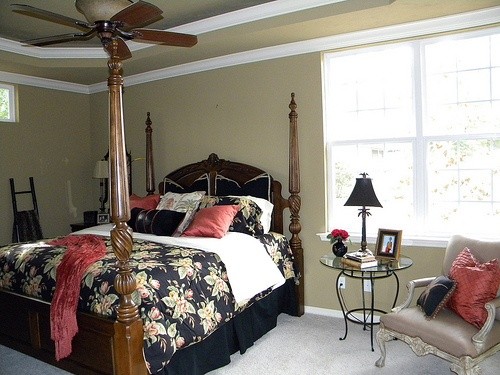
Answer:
[9,0,198,59]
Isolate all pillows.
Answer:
[447,247,500,328]
[127,191,274,238]
[416,274,459,321]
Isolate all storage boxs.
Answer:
[83,211,98,224]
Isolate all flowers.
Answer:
[327,229,349,244]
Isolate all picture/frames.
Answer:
[374,229,402,261]
[97,214,110,223]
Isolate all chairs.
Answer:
[375,234,500,375]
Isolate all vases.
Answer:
[332,238,348,257]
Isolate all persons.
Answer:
[385,237,392,253]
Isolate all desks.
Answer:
[320,254,413,352]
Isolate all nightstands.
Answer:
[70,224,100,232]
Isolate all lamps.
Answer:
[93,161,108,214]
[344,172,383,256]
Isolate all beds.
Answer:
[0,36,305,375]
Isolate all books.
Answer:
[340,252,378,269]
